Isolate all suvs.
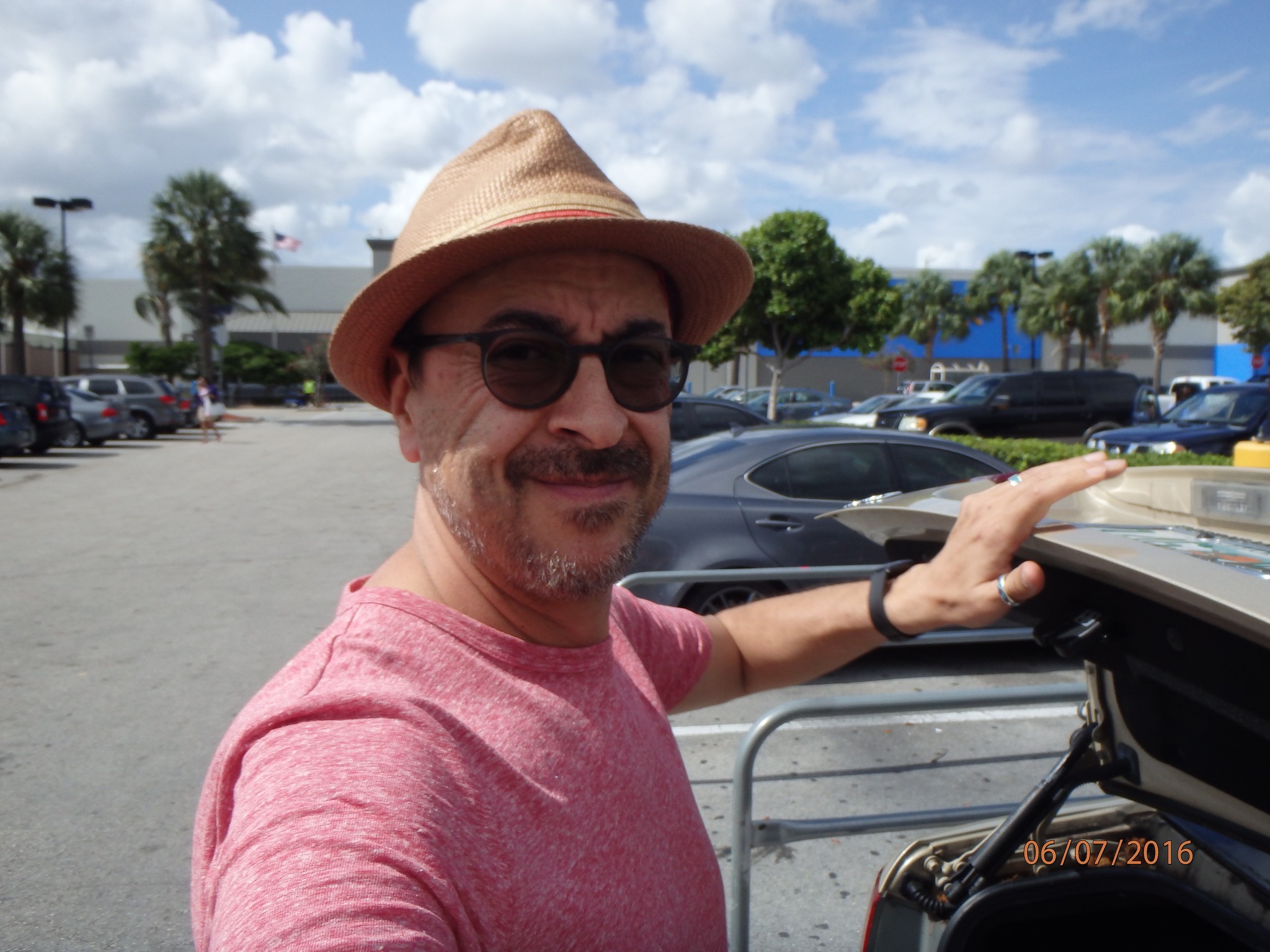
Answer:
[1088,384,1270,456]
[875,370,1139,445]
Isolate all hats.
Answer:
[330,108,755,414]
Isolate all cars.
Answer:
[0,372,191,455]
[612,420,1037,657]
[712,386,850,420]
[667,396,776,440]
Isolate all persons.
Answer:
[191,378,222,444]
[192,108,1130,952]
[303,376,316,407]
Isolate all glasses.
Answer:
[430,327,704,413]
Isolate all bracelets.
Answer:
[865,560,923,644]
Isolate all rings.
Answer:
[997,573,1021,609]
[1007,473,1021,487]
